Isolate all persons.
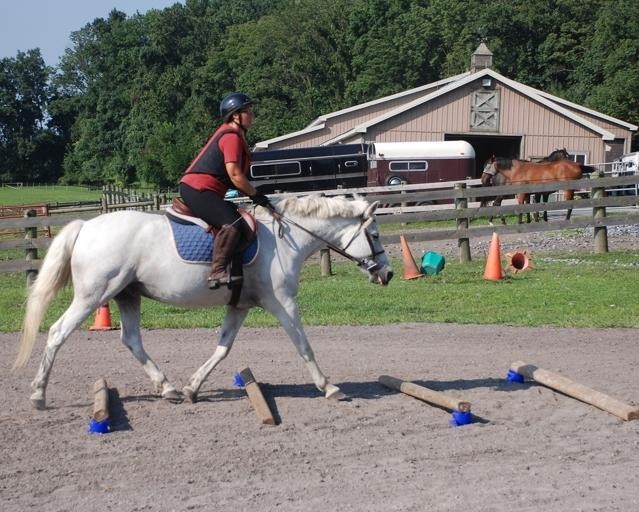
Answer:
[179,93,270,290]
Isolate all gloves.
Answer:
[249,189,270,206]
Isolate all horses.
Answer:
[11,193,393,411]
[481,147,597,224]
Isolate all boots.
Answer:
[207,224,245,289]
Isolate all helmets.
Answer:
[219,92,256,119]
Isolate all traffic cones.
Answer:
[505,249,533,275]
[400,236,425,280]
[90,303,115,330]
[485,231,501,282]
